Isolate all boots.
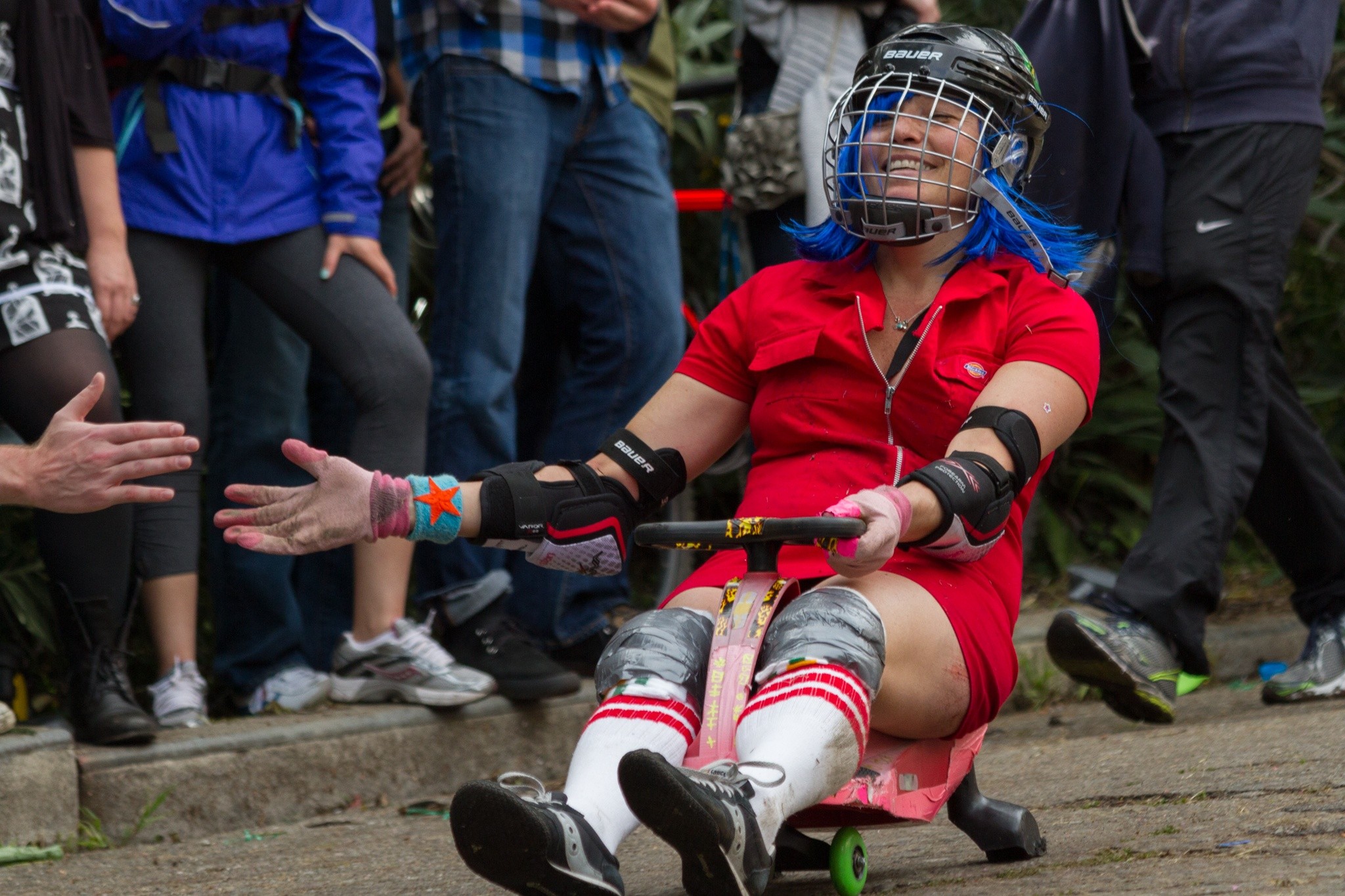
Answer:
[56,575,157,747]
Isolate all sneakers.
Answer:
[245,661,332,716]
[146,657,207,726]
[1261,612,1345,704]
[328,617,496,706]
[617,748,786,896]
[1046,611,1176,726]
[448,772,624,896]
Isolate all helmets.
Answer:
[821,22,1050,247]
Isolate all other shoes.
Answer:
[430,612,582,701]
[546,602,642,678]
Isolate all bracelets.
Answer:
[407,471,462,544]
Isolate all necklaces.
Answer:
[883,297,932,330]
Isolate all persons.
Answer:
[2,2,1345,749]
[214,18,1096,896]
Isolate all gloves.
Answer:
[214,439,414,556]
[816,484,910,579]
